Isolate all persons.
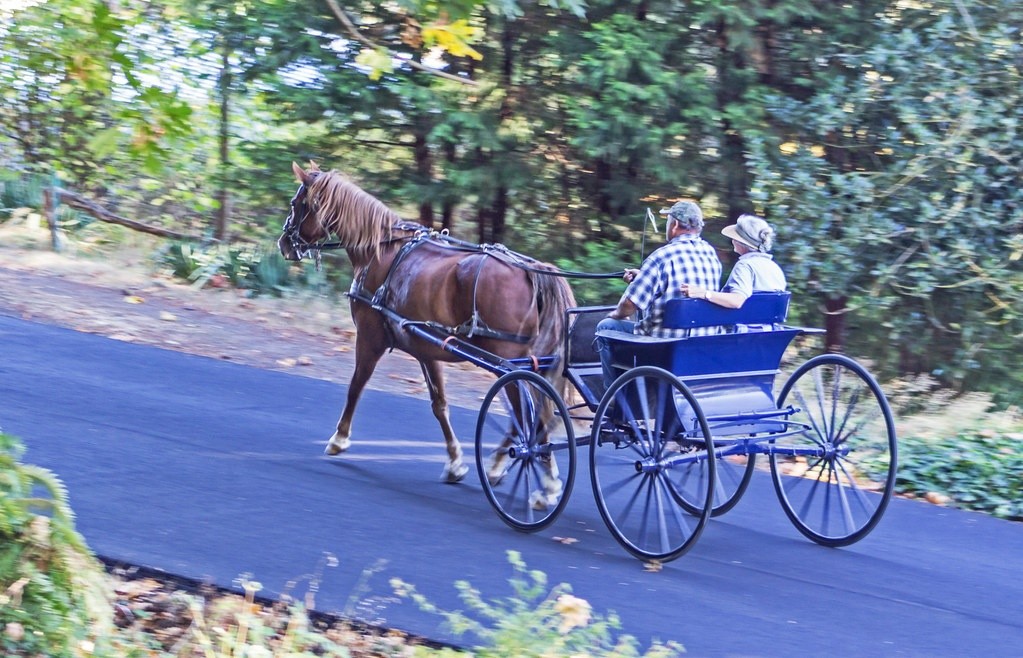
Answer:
[597,201,722,407]
[679,214,787,309]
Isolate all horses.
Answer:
[276,159,578,512]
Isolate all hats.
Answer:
[720,214,774,255]
[659,200,705,227]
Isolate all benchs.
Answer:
[594,290,828,389]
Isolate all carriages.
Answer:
[276,161,899,564]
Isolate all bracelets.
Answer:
[705,289,711,301]
